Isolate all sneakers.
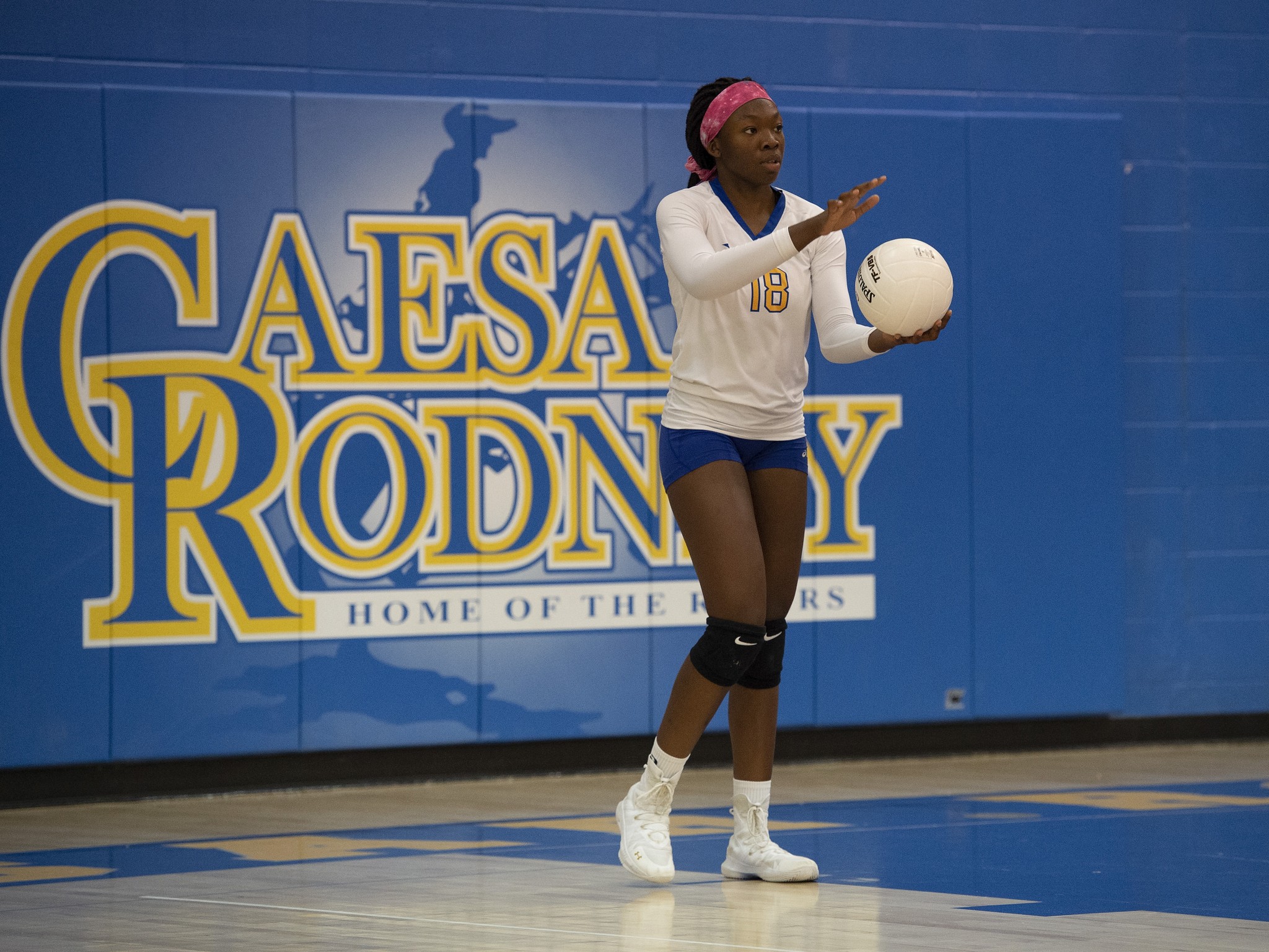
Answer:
[615,757,681,882]
[722,793,819,883]
[719,882,820,931]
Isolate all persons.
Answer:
[613,75,952,880]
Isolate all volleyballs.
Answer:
[855,237,954,338]
